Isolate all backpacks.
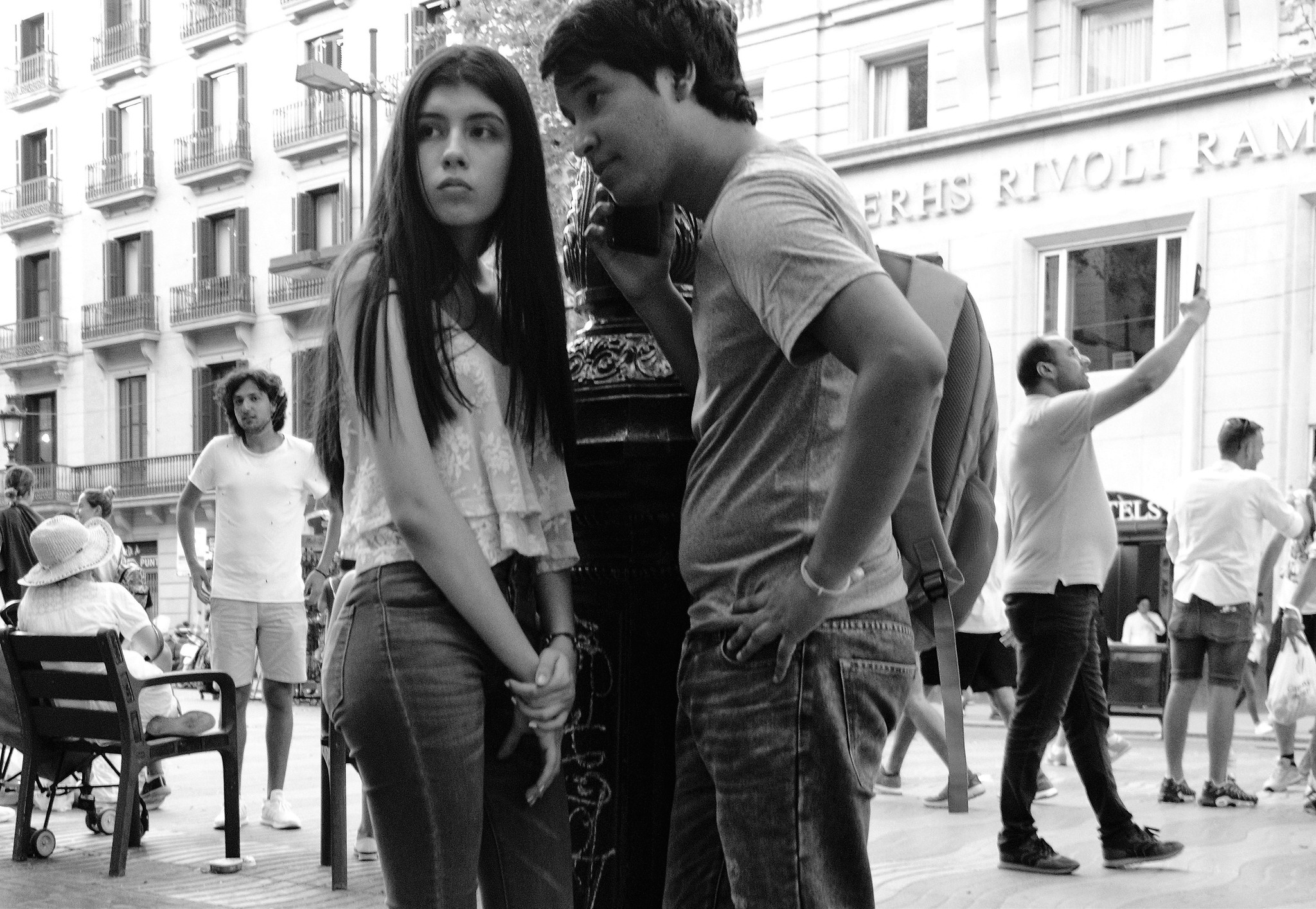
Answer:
[720,153,1000,654]
[113,545,150,611]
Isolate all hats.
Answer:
[17,514,115,587]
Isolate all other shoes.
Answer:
[923,773,985,808]
[1263,757,1302,793]
[874,768,903,795]
[353,837,379,860]
[1033,772,1057,800]
[1255,723,1273,735]
[1302,786,1316,813]
[259,788,302,829]
[214,794,247,829]
[146,710,216,737]
[1041,750,1067,767]
[139,774,171,799]
[1107,735,1130,763]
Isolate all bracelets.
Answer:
[315,569,327,578]
[800,554,851,595]
[544,632,578,653]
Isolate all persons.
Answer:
[176,371,373,860]
[323,40,587,909]
[997,291,1212,878]
[1119,595,1169,651]
[1158,418,1316,812]
[0,463,52,633]
[13,513,217,814]
[1230,596,1271,739]
[541,0,949,909]
[881,481,1131,811]
[56,488,146,654]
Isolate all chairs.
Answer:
[0,623,246,893]
[321,572,367,894]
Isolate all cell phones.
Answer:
[602,197,663,257]
[1194,264,1202,297]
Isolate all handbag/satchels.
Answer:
[1264,603,1316,725]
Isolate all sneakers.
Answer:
[1102,823,1183,867]
[1198,775,1258,809]
[1157,776,1196,805]
[996,833,1080,874]
